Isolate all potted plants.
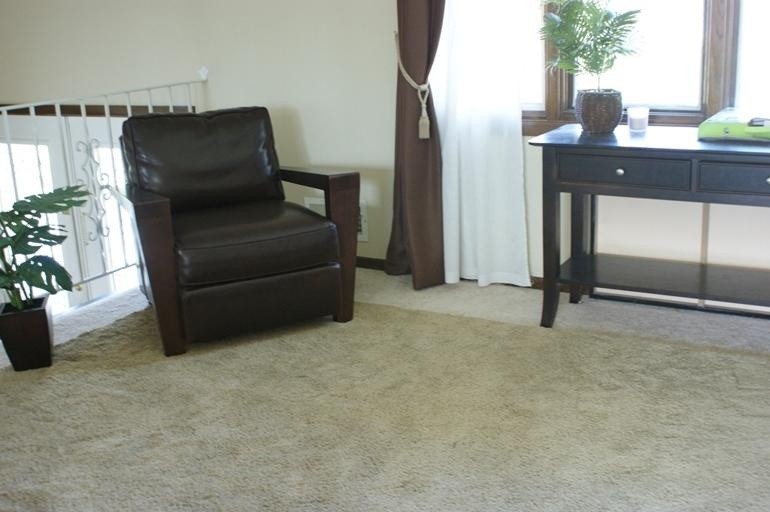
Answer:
[540,1,641,134]
[0,184,91,372]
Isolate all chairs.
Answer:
[120,132,360,357]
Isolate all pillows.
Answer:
[123,107,285,205]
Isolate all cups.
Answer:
[628,107,649,132]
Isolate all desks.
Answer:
[528,122,770,329]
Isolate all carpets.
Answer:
[1,301,769,512]
